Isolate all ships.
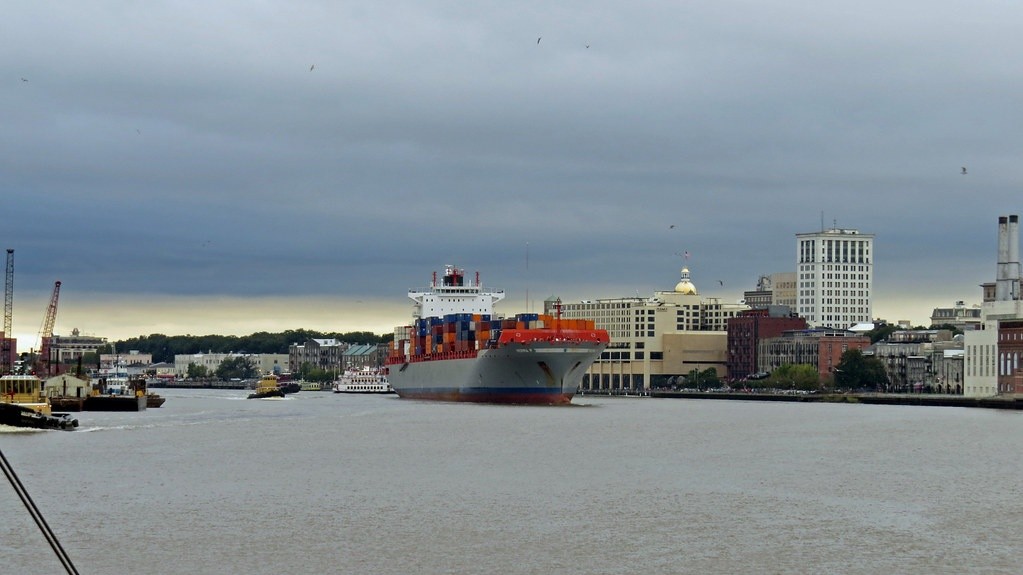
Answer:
[382,263,611,404]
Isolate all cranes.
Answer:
[2,249,15,375]
[34,280,61,370]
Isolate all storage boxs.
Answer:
[381,313,609,365]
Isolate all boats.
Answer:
[0,371,165,430]
[331,364,396,394]
[246,372,321,399]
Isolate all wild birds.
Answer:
[585,44,590,48]
[960,167,967,175]
[310,64,314,71]
[537,37,541,43]
[719,280,722,285]
[670,224,678,229]
